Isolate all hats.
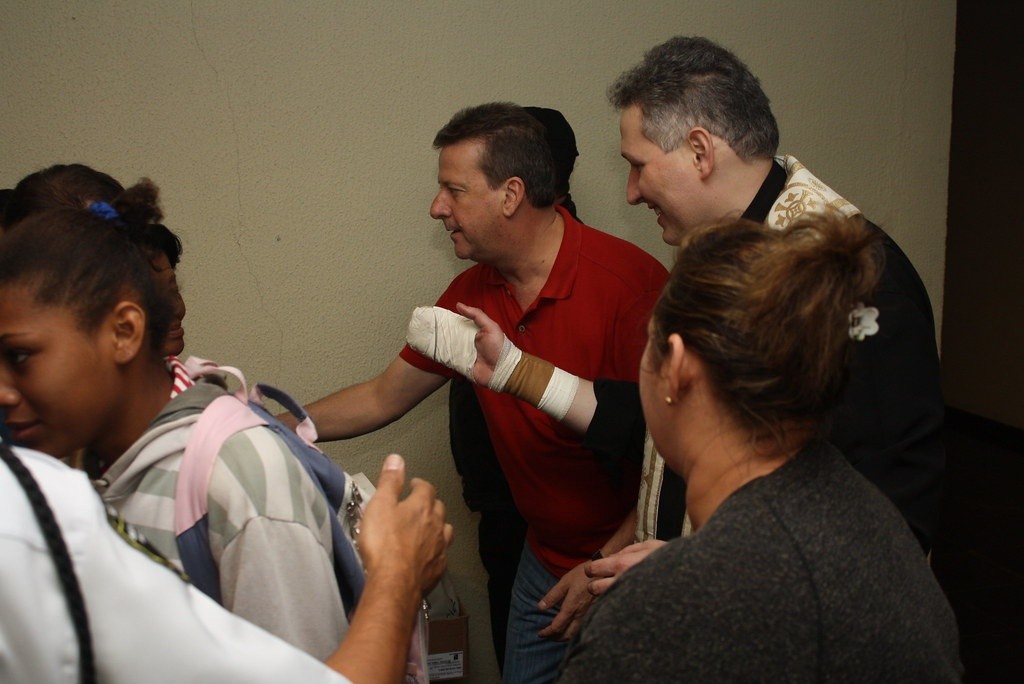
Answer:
[523,107,580,163]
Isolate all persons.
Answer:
[0,165,367,684]
[272,100,670,684]
[0,446,454,683]
[403,38,946,644]
[558,206,972,684]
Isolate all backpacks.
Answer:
[175,366,431,684]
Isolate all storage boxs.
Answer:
[427,602,471,684]
